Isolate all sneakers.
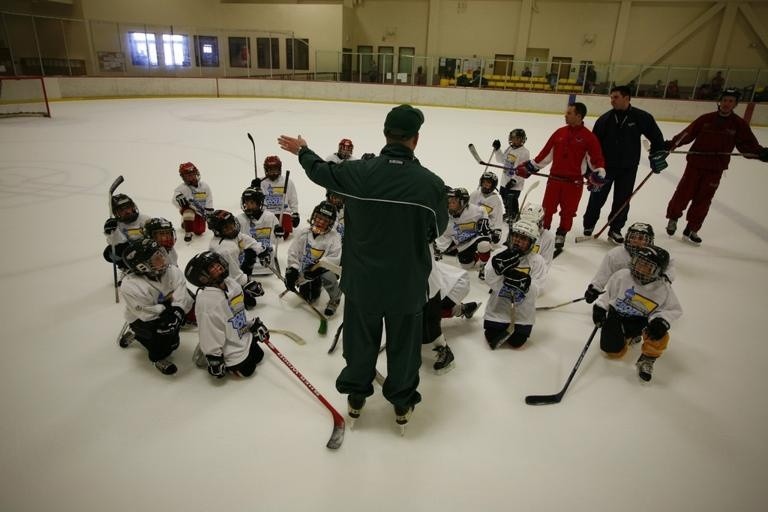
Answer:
[324,299,340,316]
[584,228,594,235]
[181,222,184,228]
[152,359,178,375]
[119,326,136,348]
[608,228,624,243]
[243,290,257,306]
[394,405,415,424]
[667,218,678,235]
[479,263,487,279]
[196,351,208,369]
[284,232,289,240]
[507,213,520,223]
[503,213,510,218]
[184,233,193,242]
[628,336,642,345]
[461,301,477,320]
[432,346,454,370]
[306,294,321,303]
[683,224,702,243]
[555,228,566,248]
[118,272,129,286]
[637,353,658,381]
[348,393,366,418]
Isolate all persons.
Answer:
[581,87,669,246]
[440,61,488,88]
[434,188,493,281]
[492,130,532,225]
[184,251,263,380]
[546,67,560,93]
[278,103,448,427]
[103,192,149,272]
[577,62,726,100]
[661,88,767,246]
[420,260,480,369]
[236,186,287,275]
[482,221,548,350]
[592,246,683,385]
[585,222,677,303]
[250,156,299,240]
[468,171,506,248]
[205,210,272,311]
[521,65,531,78]
[172,163,214,244]
[516,103,606,251]
[284,199,343,318]
[325,186,345,238]
[116,236,187,376]
[367,61,378,83]
[129,218,178,269]
[324,136,359,166]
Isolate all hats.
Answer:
[384,105,424,135]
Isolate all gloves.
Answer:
[304,266,330,280]
[291,212,300,228]
[492,139,501,151]
[104,218,118,235]
[648,150,670,174]
[251,178,262,187]
[644,317,670,341]
[755,145,768,162]
[250,317,270,343]
[156,306,186,335]
[586,168,606,193]
[274,224,285,238]
[434,249,443,261]
[203,208,214,223]
[258,246,271,268]
[476,219,491,236]
[162,297,172,309]
[593,304,607,328]
[284,267,300,292]
[585,284,600,304]
[665,139,674,150]
[205,354,226,378]
[176,193,190,207]
[492,229,502,244]
[492,248,521,276]
[242,280,264,297]
[505,178,517,190]
[503,269,531,294]
[515,159,540,179]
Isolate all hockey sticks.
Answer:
[248,132,257,178]
[327,321,344,355]
[268,328,305,345]
[517,181,541,220]
[641,140,764,159]
[262,338,345,448]
[526,322,602,405]
[535,290,607,311]
[469,144,587,185]
[493,214,514,350]
[108,175,124,302]
[375,340,387,385]
[274,171,290,274]
[266,262,327,334]
[575,132,689,243]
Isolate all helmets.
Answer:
[507,219,540,257]
[307,200,337,235]
[264,156,281,180]
[207,210,241,244]
[144,217,177,252]
[242,187,266,220]
[338,139,354,160]
[185,250,230,287]
[508,129,527,149]
[630,244,671,285]
[520,203,545,225]
[179,162,201,186]
[111,194,140,225]
[624,222,654,256]
[717,88,741,114]
[479,173,498,195]
[122,239,171,277]
[447,188,470,217]
